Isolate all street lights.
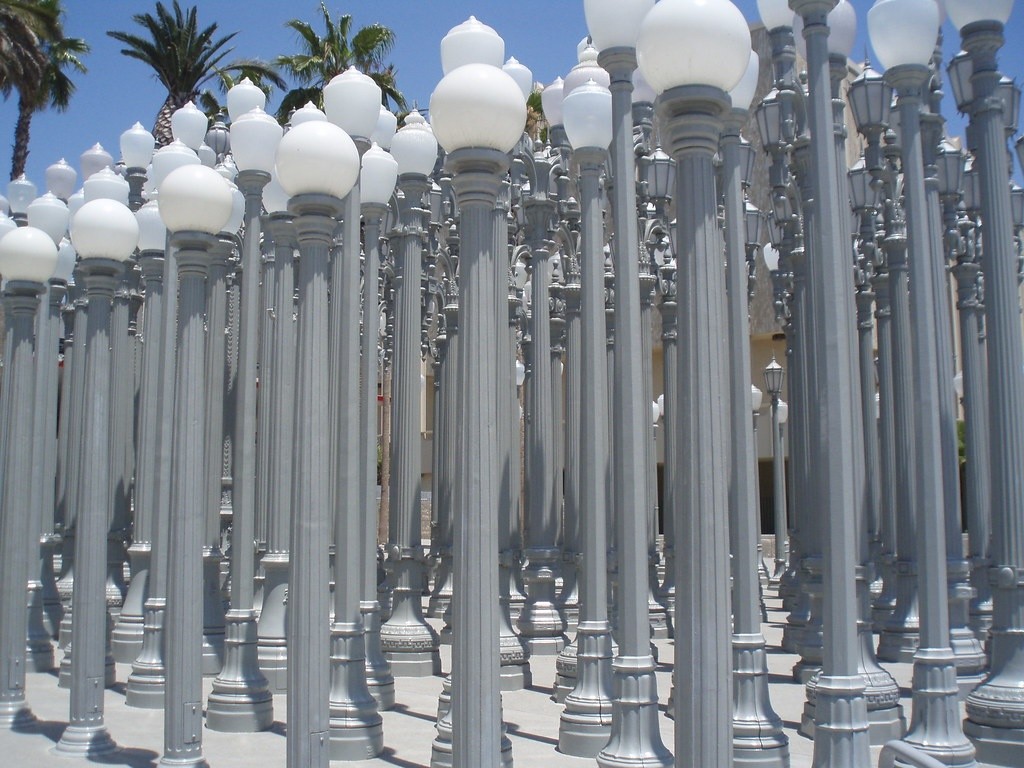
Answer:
[1,0,1021,768]
